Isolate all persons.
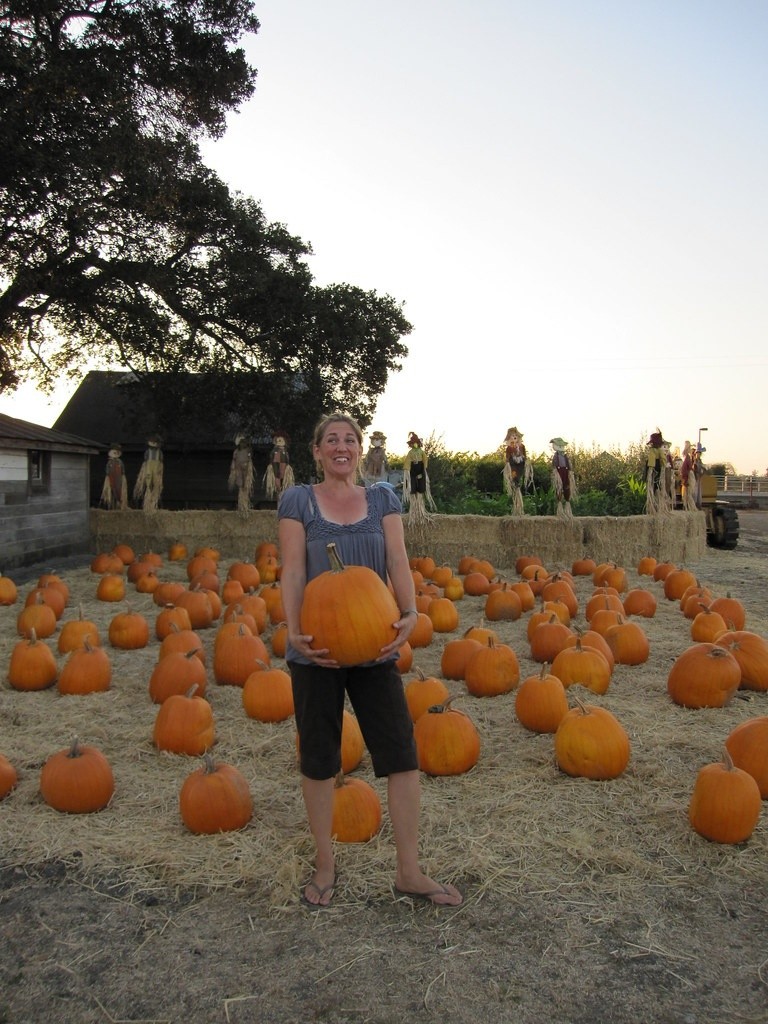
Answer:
[403,432,437,527]
[228,437,257,512]
[133,432,163,511]
[101,442,132,511]
[502,427,533,517]
[277,412,462,906]
[263,431,296,500]
[642,431,706,517]
[550,438,578,519]
[363,431,390,487]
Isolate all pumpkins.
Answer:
[341,710,362,773]
[331,773,382,842]
[0,542,297,836]
[299,543,400,665]
[390,555,768,843]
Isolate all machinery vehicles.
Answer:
[669,473,740,550]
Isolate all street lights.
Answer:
[699,428,708,442]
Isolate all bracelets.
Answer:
[402,608,418,619]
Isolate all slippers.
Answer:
[303,880,335,906]
[395,882,460,906]
